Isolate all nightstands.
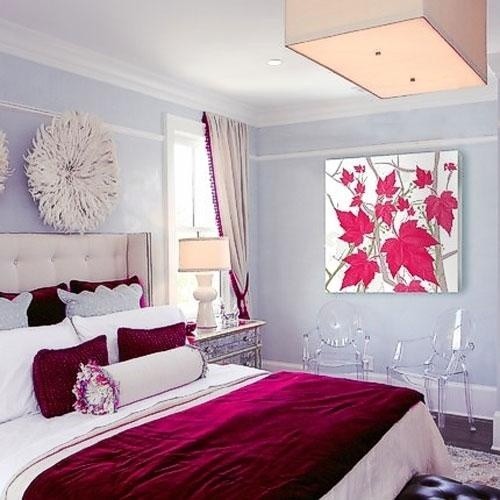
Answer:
[191,314,267,369]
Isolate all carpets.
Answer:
[444,439,499,492]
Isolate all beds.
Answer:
[1,229,456,500]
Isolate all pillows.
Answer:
[57,280,144,328]
[68,272,147,308]
[71,342,207,415]
[117,321,187,361]
[1,294,36,330]
[72,304,185,364]
[1,282,69,328]
[1,317,81,426]
[31,332,109,418]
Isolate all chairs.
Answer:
[387,306,480,430]
[300,297,373,380]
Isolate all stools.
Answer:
[396,470,500,500]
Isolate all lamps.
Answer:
[179,236,230,329]
[282,1,487,102]
